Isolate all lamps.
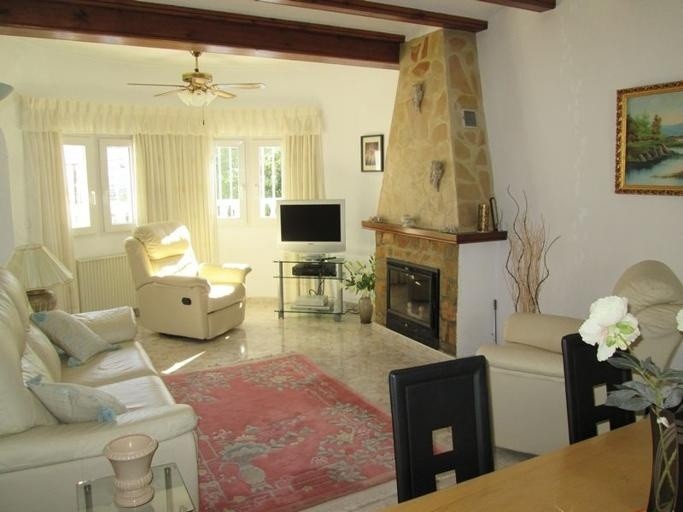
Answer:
[178,88,217,106]
[3,242,74,310]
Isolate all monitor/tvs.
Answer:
[276,199,346,258]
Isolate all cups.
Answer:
[400,215,410,228]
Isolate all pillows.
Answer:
[31,308,119,362]
[26,382,128,425]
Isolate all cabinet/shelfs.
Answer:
[273,257,357,323]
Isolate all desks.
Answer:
[380,418,653,512]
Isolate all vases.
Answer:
[101,434,159,507]
[359,295,373,324]
[648,411,683,511]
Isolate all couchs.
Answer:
[123,219,254,343]
[0,270,201,512]
[472,259,683,457]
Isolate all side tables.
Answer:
[74,461,195,512]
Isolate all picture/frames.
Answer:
[361,134,384,172]
[615,82,683,197]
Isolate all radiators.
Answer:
[76,253,138,317]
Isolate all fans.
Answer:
[127,50,265,100]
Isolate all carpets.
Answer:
[163,352,443,512]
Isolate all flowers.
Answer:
[577,293,683,431]
[338,255,374,297]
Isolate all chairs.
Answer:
[560,331,636,445]
[384,356,495,501]
